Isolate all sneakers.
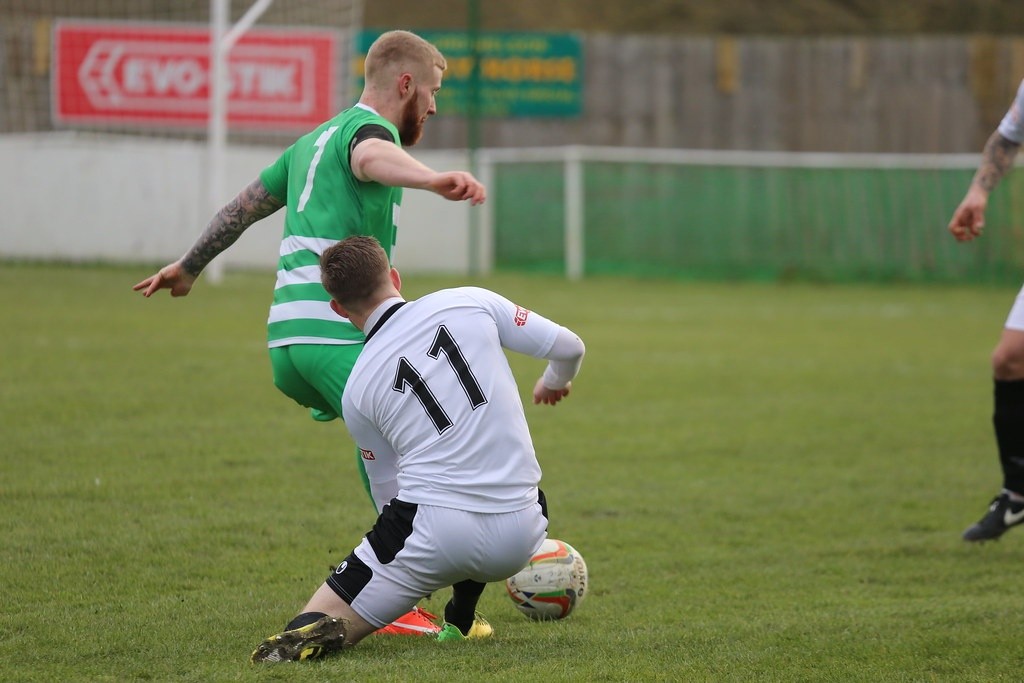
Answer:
[250,614,351,665]
[965,494,1024,541]
[372,604,443,635]
[435,609,495,643]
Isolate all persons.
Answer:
[133,30,486,636]
[948,75,1024,545]
[252,234,586,662]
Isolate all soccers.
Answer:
[504,537,589,620]
